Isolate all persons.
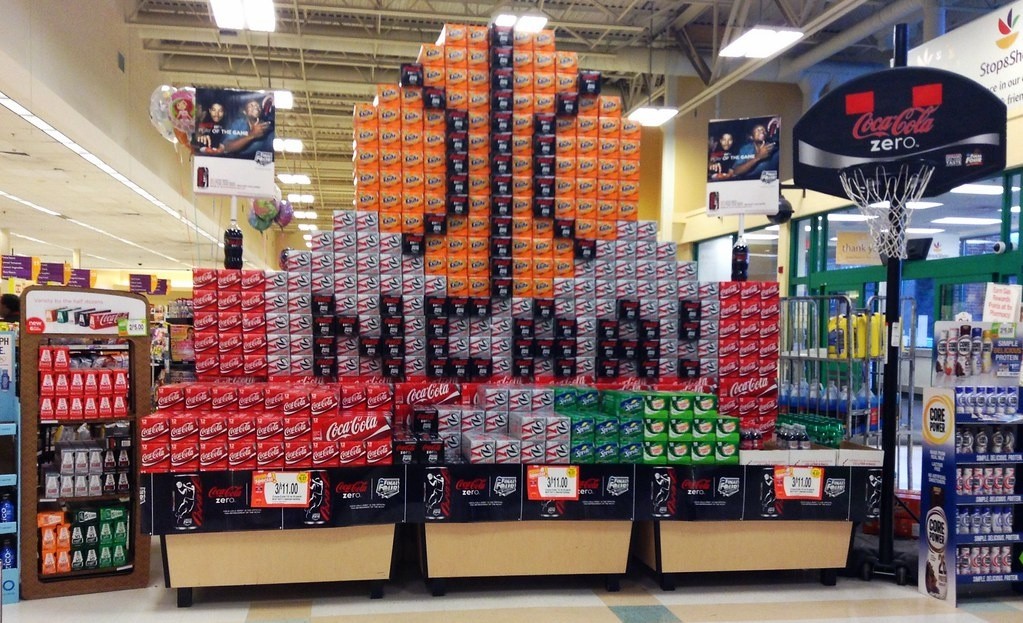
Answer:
[153,327,195,407]
[195,95,275,155]
[708,116,780,181]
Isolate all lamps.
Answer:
[245,34,293,111]
[720,22,804,60]
[488,0,547,31]
[630,2,677,125]
[209,0,277,31]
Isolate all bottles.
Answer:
[0,538,17,570]
[936,325,993,379]
[739,311,887,452]
[0,493,15,524]
[954,385,1018,575]
[922,487,948,599]
[2,370,9,390]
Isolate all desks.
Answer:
[144,464,411,607]
[410,463,639,597]
[641,443,885,594]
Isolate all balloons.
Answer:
[247,184,293,237]
[148,84,196,164]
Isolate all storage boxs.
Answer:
[33,23,781,577]
[864,490,922,540]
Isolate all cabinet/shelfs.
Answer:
[0,388,22,606]
[917,385,1023,609]
